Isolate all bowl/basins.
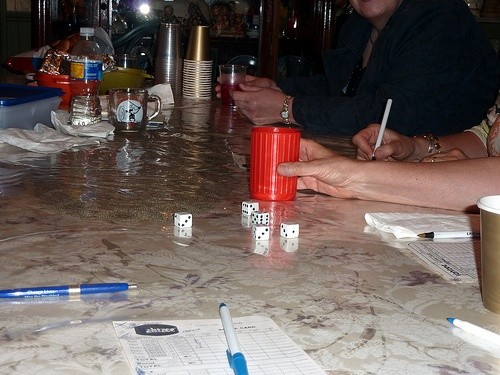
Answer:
[103,54,147,92]
[36,73,102,111]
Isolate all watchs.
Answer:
[280,94,294,125]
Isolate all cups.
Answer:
[154,23,184,101]
[475,194,500,315]
[108,88,161,136]
[219,64,246,111]
[182,26,213,101]
[248,126,301,201]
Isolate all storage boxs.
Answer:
[0,83,65,128]
[33,71,71,114]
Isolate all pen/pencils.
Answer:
[0,282,138,298]
[417,231,480,238]
[372,99,393,161]
[446,318,500,345]
[219,303,248,375]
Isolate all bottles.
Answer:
[68,27,102,126]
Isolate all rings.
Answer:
[430,157,436,163]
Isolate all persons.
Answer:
[215,0,500,134]
[276,138,500,214]
[351,94,500,163]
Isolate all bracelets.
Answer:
[412,133,441,154]
